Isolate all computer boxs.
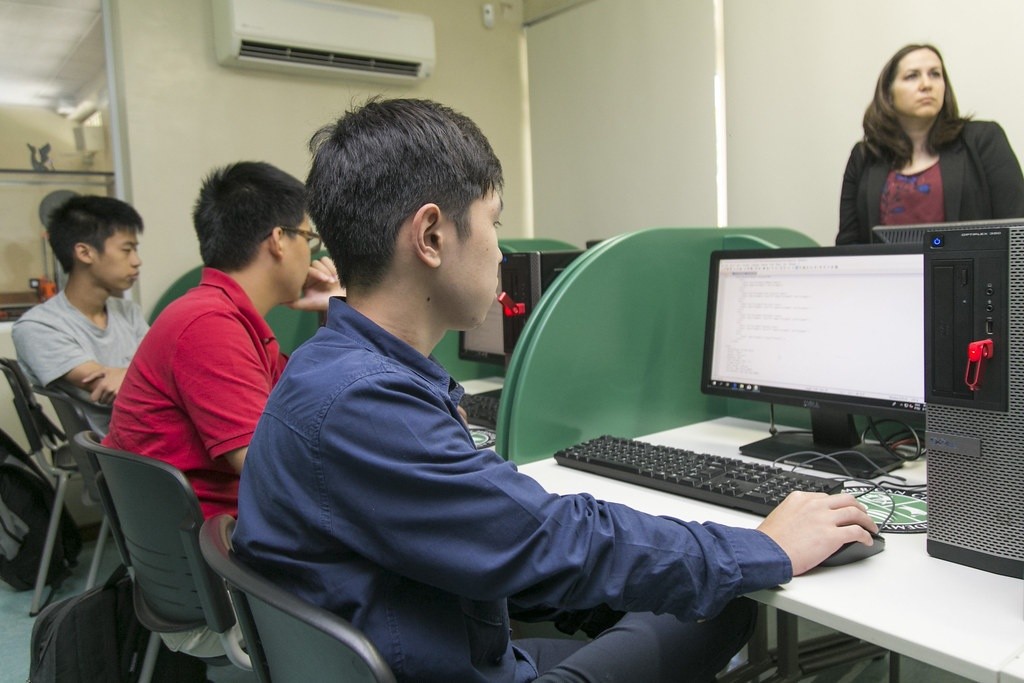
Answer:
[923,219,1024,579]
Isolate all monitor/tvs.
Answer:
[458,251,541,364]
[700,241,924,415]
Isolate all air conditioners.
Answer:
[211,3,437,82]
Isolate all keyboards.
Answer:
[553,429,844,517]
[456,386,502,430]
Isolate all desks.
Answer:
[512,416,1023,682]
[453,376,510,454]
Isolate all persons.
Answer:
[11,195,150,408]
[834,44,1022,244]
[107,160,349,523]
[231,94,883,683]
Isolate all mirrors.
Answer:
[0,0,117,305]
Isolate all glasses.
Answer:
[265,225,322,254]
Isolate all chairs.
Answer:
[198,515,396,682]
[75,429,254,683]
[0,357,109,618]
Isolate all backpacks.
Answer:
[0,429,83,591]
[29,565,213,683]
